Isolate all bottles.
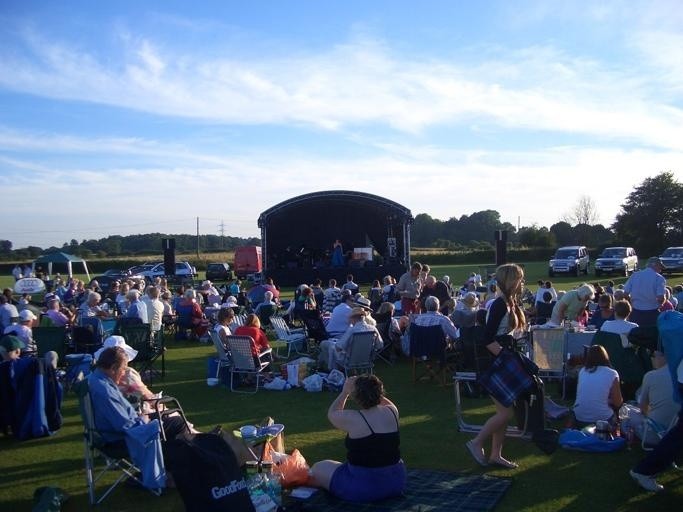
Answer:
[304,302,309,310]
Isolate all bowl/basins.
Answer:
[206,378,219,386]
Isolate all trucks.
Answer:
[234,246,263,279]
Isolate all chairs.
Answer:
[81,390,168,507]
[639,409,681,471]
[3,359,65,439]
[451,368,533,441]
[164,435,255,511]
[242,423,287,477]
[155,396,192,444]
[2,279,682,396]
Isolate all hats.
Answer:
[647,257,666,270]
[20,309,38,321]
[94,335,138,364]
[345,297,373,318]
[0,335,27,351]
[202,280,213,286]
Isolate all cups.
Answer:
[596,419,609,440]
[260,460,273,475]
[245,460,258,478]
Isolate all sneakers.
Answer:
[630,470,664,491]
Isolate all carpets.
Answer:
[281,467,512,510]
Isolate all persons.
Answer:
[332,239,344,266]
[0,256,683,504]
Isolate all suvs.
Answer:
[548,245,589,278]
[107,264,154,284]
[594,246,638,276]
[658,247,682,276]
[134,262,197,287]
[206,262,232,281]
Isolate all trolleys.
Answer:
[154,397,256,512]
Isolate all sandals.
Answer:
[465,439,519,469]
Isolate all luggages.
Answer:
[518,374,545,434]
